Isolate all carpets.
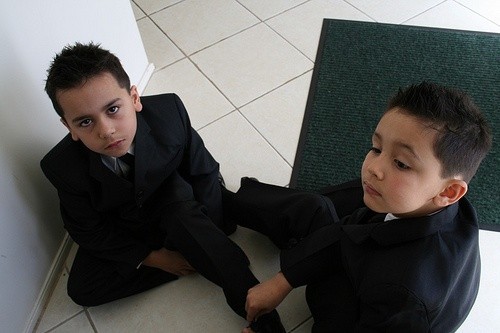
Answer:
[288,17,500,233]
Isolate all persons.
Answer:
[40,42,286,333]
[236,80,491,333]
